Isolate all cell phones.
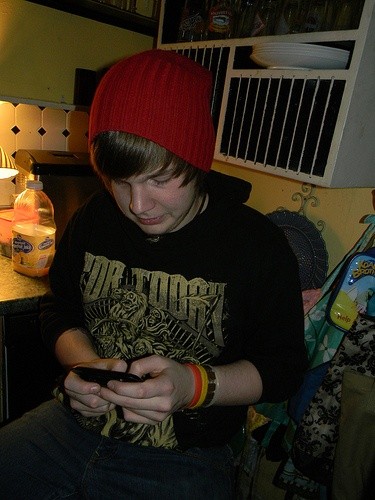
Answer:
[71,366,139,388]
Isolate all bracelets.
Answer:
[182,362,220,409]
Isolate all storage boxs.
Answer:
[12,149,99,251]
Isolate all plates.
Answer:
[249,43,350,71]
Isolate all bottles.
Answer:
[207,0,235,40]
[11,179,56,278]
[178,0,205,42]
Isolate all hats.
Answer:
[88,50,216,173]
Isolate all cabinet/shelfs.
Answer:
[152,0,375,188]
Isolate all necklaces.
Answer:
[142,190,206,242]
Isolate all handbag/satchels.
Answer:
[326,252,375,336]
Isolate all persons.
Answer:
[0,49,308,500]
